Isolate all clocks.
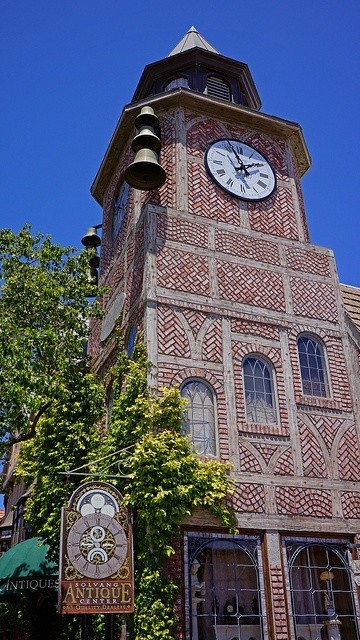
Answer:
[204,138,276,201]
